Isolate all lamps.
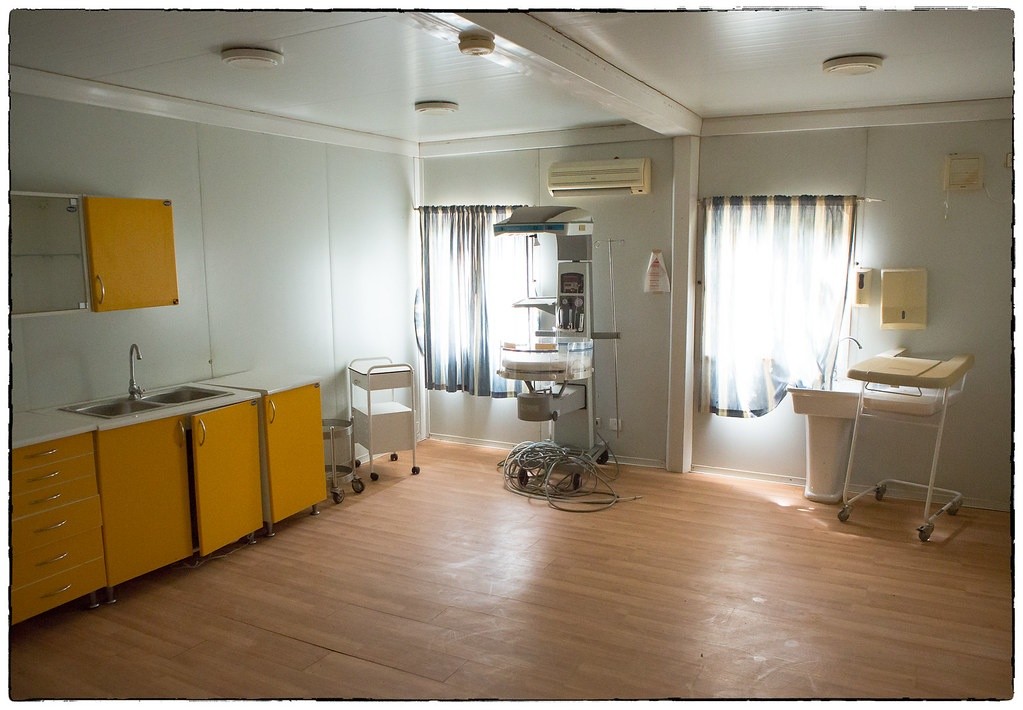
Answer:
[221,48,286,70]
[415,101,460,115]
[822,56,884,75]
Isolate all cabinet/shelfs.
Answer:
[12,370,107,627]
[197,369,327,536]
[11,190,179,318]
[92,400,263,605]
[347,357,420,480]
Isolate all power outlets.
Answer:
[1006,154,1012,167]
[596,417,601,430]
[609,419,621,431]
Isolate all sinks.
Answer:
[785,380,860,420]
[76,399,165,419]
[142,388,235,407]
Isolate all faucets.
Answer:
[838,337,863,351]
[128,343,146,399]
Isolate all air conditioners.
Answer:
[546,157,652,197]
